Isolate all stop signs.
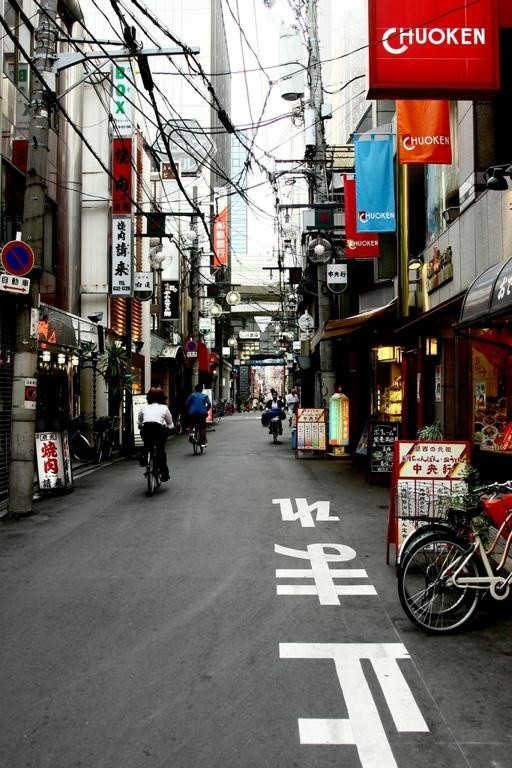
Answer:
[0,240,34,276]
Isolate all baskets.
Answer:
[484,494,512,542]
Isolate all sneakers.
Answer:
[201,443,208,448]
[139,456,146,466]
[189,433,194,442]
[162,475,169,481]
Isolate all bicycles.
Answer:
[188,424,204,456]
[270,417,280,441]
[69,411,119,465]
[396,480,512,636]
[143,446,162,495]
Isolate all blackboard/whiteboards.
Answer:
[368,423,401,475]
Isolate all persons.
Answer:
[242,388,300,435]
[185,384,211,448]
[138,387,174,481]
